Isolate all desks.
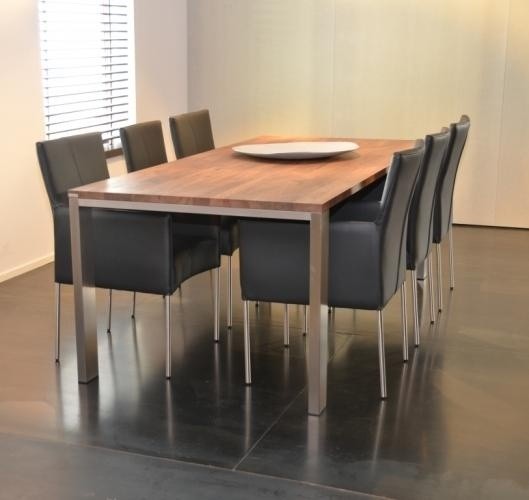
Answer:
[65,133,415,417]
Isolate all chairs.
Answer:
[169,108,262,327]
[238,138,427,397]
[437,113,471,314]
[119,118,225,343]
[35,131,223,384]
[329,125,451,348]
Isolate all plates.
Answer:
[231,142,360,160]
